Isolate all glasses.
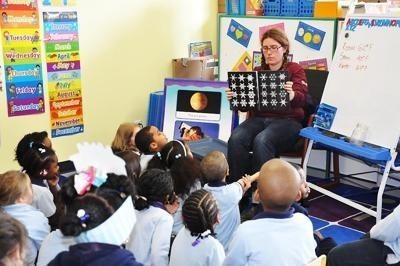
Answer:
[262,45,282,51]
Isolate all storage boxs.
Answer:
[280,0,318,18]
[262,0,281,16]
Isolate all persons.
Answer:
[327,202,400,266]
[0,171,50,266]
[43,195,144,266]
[241,164,311,229]
[111,122,143,163]
[106,151,141,206]
[60,175,97,208]
[19,142,62,227]
[223,158,318,266]
[227,29,308,179]
[168,191,226,266]
[15,131,54,178]
[198,151,261,251]
[180,125,205,142]
[153,141,193,172]
[0,216,26,266]
[117,171,180,266]
[170,158,200,259]
[135,126,168,181]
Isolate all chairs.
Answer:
[248,69,330,168]
